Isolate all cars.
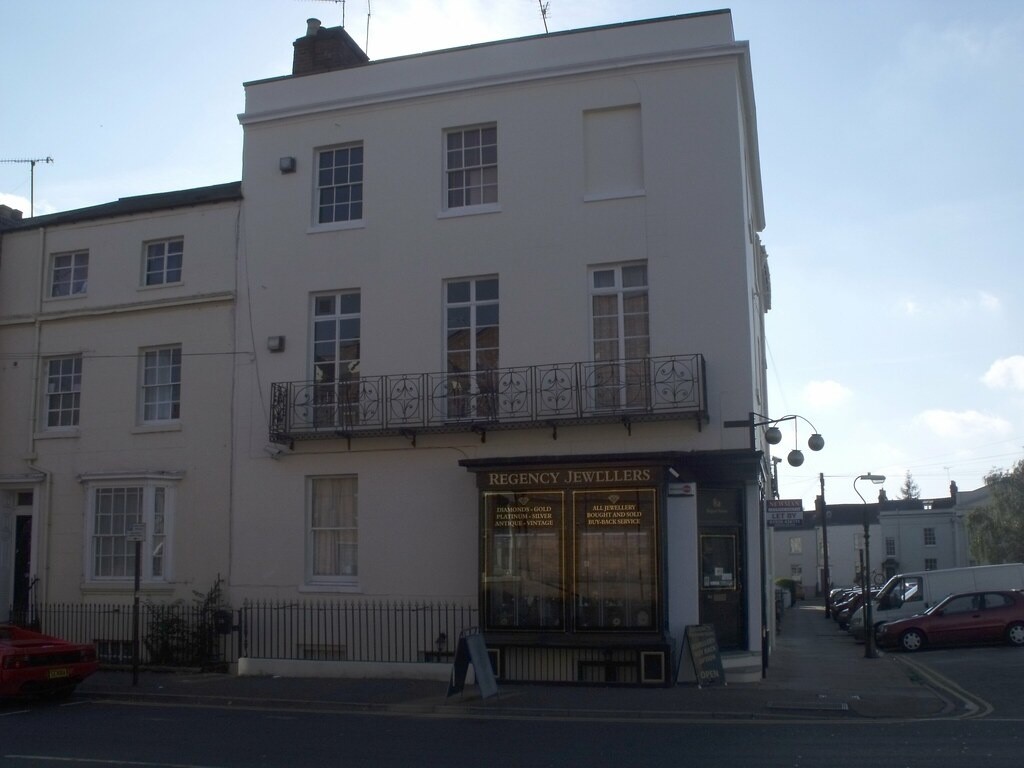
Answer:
[873,588,1024,654]
[829,582,918,628]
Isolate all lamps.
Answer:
[280,156,296,174]
[266,335,283,352]
[750,414,825,465]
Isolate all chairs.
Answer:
[972,598,979,609]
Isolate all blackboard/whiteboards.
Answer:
[677,623,726,684]
[447,632,500,698]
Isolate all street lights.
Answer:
[853,471,886,657]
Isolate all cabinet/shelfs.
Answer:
[494,535,653,628]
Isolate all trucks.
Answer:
[849,563,1024,641]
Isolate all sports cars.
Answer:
[0,624,101,701]
